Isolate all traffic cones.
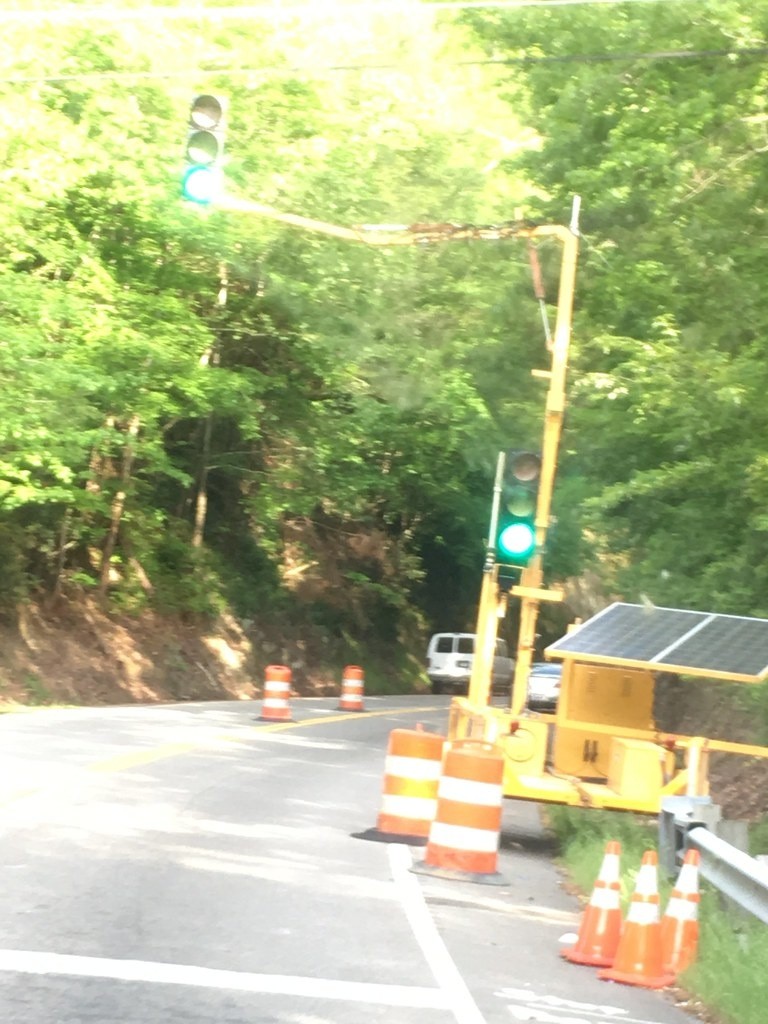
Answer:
[558,837,625,969]
[661,848,702,974]
[597,849,677,990]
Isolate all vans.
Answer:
[423,632,518,695]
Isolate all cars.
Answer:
[525,661,568,713]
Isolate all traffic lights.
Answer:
[182,93,234,206]
[491,449,544,572]
